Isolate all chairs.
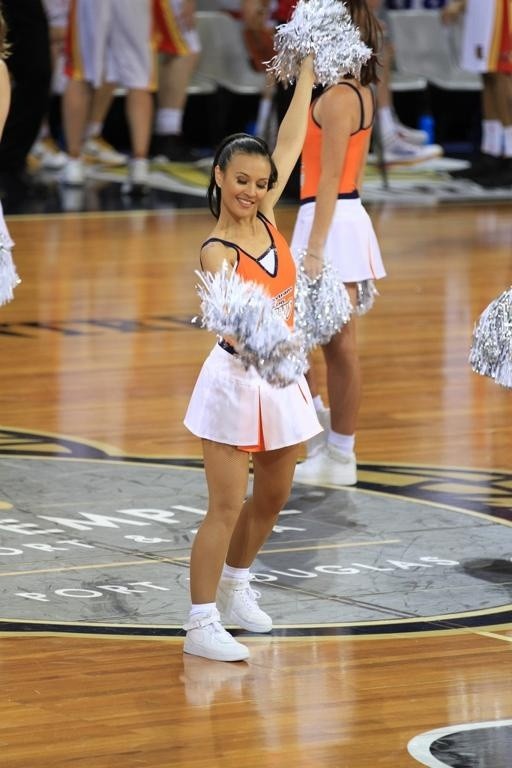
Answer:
[184,12,268,94]
[380,9,483,93]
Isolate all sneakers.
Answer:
[215,570,274,634]
[391,119,430,144]
[119,158,151,198]
[152,133,216,172]
[303,406,333,457]
[182,604,252,665]
[79,136,128,169]
[290,442,358,486]
[373,134,444,171]
[54,157,87,188]
[473,151,512,189]
[28,137,68,172]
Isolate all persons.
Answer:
[281,0,386,486]
[0,1,512,196]
[2,22,22,316]
[184,50,317,662]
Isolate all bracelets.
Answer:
[307,249,328,266]
